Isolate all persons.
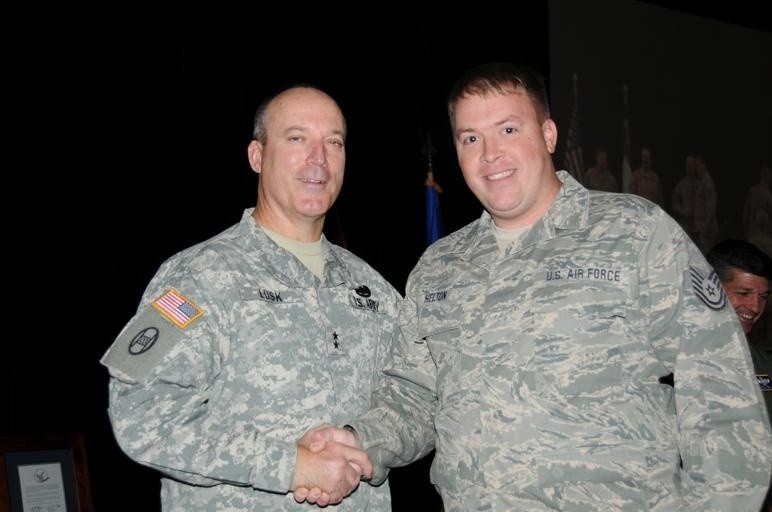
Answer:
[672,156,719,249]
[298,61,771,512]
[741,162,770,253]
[702,239,772,426]
[582,146,617,193]
[99,88,443,512]
[628,147,665,206]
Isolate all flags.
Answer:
[421,173,443,245]
[620,108,635,192]
[562,92,584,183]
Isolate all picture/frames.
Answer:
[0,430,94,512]
